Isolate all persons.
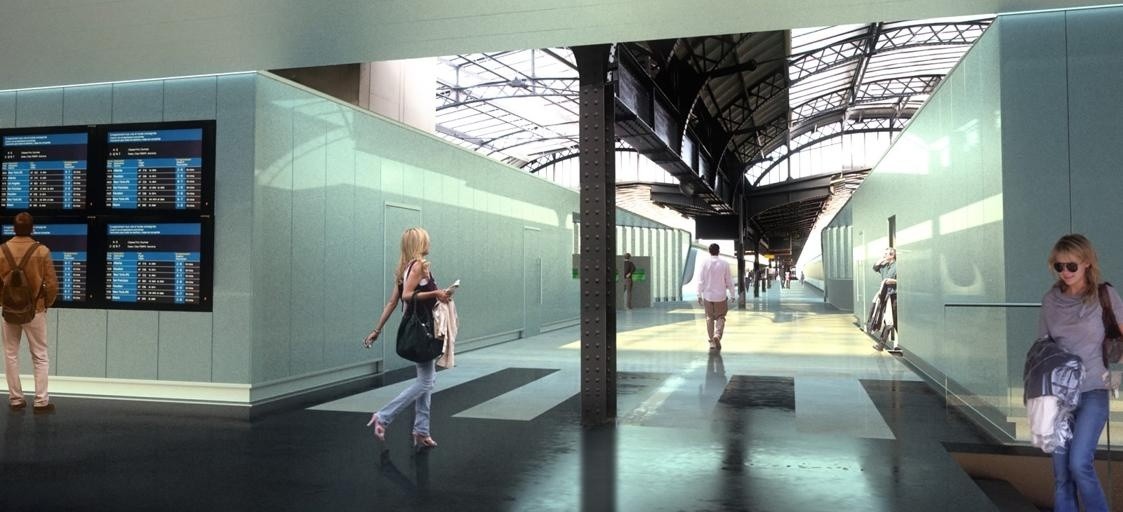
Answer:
[697,243,735,350]
[744,264,805,293]
[872,248,901,352]
[1038,233,1123,512]
[0,212,57,414]
[364,227,451,448]
[624,253,635,309]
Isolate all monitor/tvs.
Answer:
[0,212,94,309]
[95,120,213,219]
[768,235,792,250]
[696,214,739,240]
[0,127,95,217]
[734,239,755,251]
[95,214,213,313]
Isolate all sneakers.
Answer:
[33,403,54,412]
[873,345,883,351]
[11,400,27,410]
[709,336,721,350]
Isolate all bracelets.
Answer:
[374,329,381,333]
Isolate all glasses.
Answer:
[1054,261,1084,272]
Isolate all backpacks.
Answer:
[0,243,45,324]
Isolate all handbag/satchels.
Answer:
[1098,282,1123,370]
[396,290,444,362]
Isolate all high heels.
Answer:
[366,413,386,441]
[412,432,437,447]
